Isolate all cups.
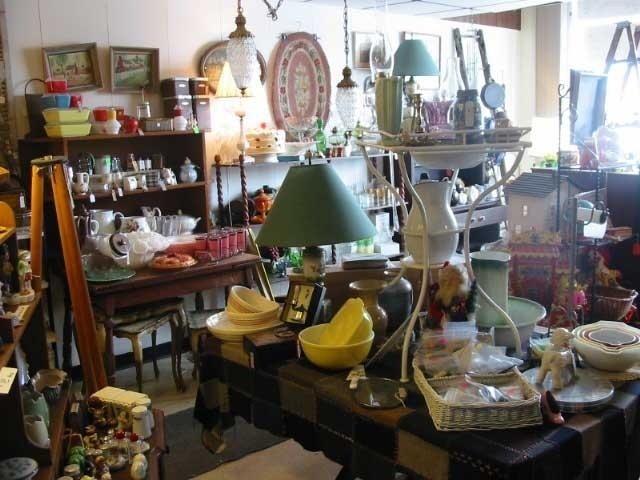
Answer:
[472,253,510,323]
[97,230,152,264]
[94,155,111,175]
[196,228,245,260]
[91,209,124,236]
[179,157,197,183]
[156,214,180,237]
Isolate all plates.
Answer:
[206,309,284,341]
[86,268,137,281]
[524,367,613,414]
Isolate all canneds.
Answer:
[194,228,248,262]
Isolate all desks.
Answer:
[55,253,259,389]
[192,322,640,479]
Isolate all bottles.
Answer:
[116,429,140,464]
[137,102,151,131]
[103,109,121,134]
[133,406,152,439]
[315,119,326,156]
[173,107,187,131]
[346,177,396,207]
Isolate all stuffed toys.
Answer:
[556,271,589,307]
[590,247,621,287]
[18,249,32,297]
[428,262,478,329]
[549,305,573,331]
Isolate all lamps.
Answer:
[335,66,360,140]
[383,39,440,147]
[228,0,256,230]
[216,60,261,154]
[254,163,379,278]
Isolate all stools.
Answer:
[184,309,226,379]
[114,312,179,394]
[72,296,187,401]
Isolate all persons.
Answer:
[117,55,125,72]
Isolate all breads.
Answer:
[155,251,192,264]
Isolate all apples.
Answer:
[124,118,138,133]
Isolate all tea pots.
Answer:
[141,206,161,234]
[178,210,200,235]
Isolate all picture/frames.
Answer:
[400,32,442,92]
[353,30,385,70]
[109,45,162,95]
[41,42,103,92]
[199,40,266,93]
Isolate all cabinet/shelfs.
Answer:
[213,152,407,282]
[18,129,210,256]
[400,152,508,251]
[0,81,165,480]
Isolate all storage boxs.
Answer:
[161,77,212,129]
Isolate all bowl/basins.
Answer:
[224,286,279,324]
[298,297,375,371]
[480,295,546,350]
[571,321,640,372]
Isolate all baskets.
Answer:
[585,283,640,322]
[413,349,544,431]
